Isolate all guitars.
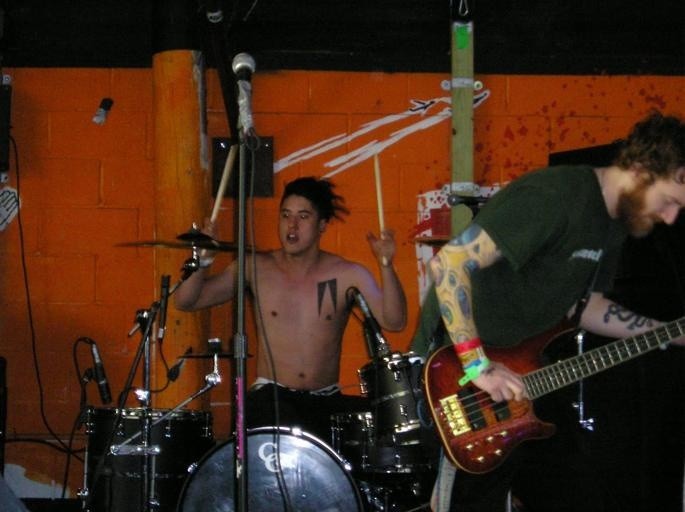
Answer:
[426,316,685,474]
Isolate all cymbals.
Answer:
[114,233,273,256]
[178,350,252,357]
[410,234,453,246]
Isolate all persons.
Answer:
[411,106,685,512]
[176,175,410,459]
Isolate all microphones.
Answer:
[231,52,257,136]
[158,274,171,342]
[353,288,392,359]
[447,194,488,206]
[90,343,112,405]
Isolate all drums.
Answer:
[176,426,399,511]
[358,350,431,448]
[90,406,213,511]
[331,411,442,508]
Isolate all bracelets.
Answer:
[455,346,487,366]
[453,336,484,356]
[462,352,487,371]
[199,255,217,270]
[458,356,491,387]
